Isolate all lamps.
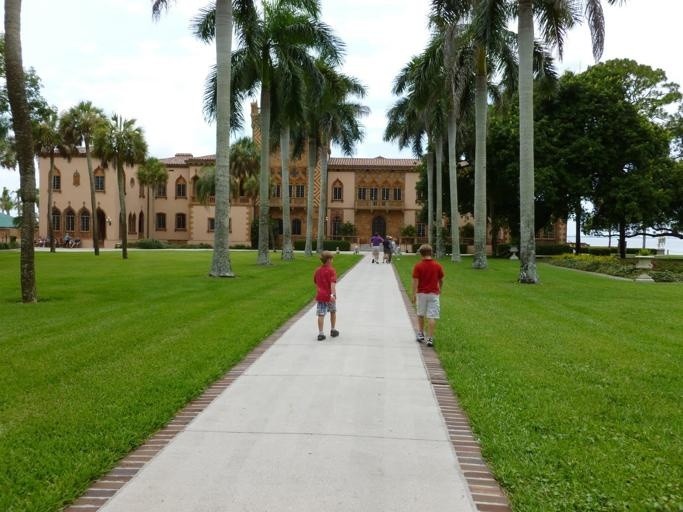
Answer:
[106,217,112,225]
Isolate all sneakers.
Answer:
[318,335,326,340]
[416,332,433,346]
[331,330,339,337]
[372,259,379,264]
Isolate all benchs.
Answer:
[58,242,79,248]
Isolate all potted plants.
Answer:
[635,249,656,282]
[508,240,520,260]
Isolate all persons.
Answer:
[369,232,393,263]
[411,243,445,346]
[312,251,339,340]
[37,232,81,248]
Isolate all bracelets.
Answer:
[330,294,336,296]
